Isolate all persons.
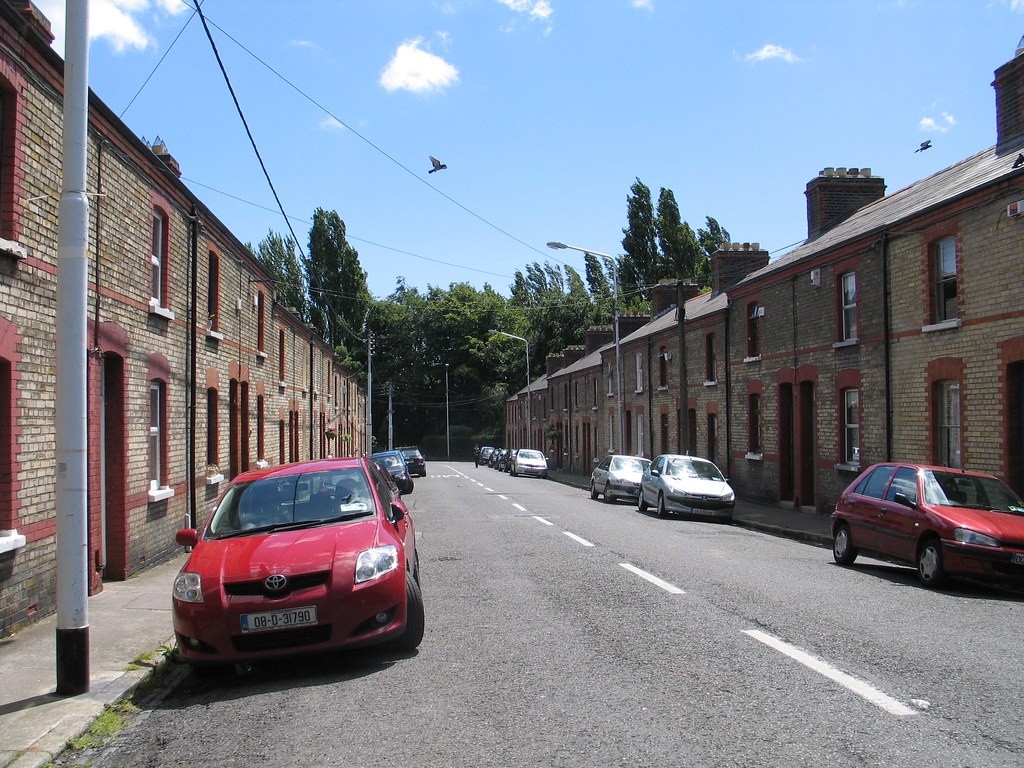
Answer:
[474,444,479,469]
[324,478,371,514]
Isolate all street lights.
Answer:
[445,364,449,458]
[547,242,623,455]
[489,329,531,448]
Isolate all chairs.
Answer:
[335,479,357,505]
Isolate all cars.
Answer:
[368,450,414,494]
[480,447,493,463]
[488,449,500,468]
[482,448,495,464]
[398,448,426,476]
[590,455,653,503]
[639,454,736,520]
[172,457,424,677]
[499,449,518,472]
[495,449,508,470]
[830,463,1023,582]
[511,448,549,479]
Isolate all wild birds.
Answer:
[914,140,932,153]
[427,155,448,174]
[1012,154,1024,169]
